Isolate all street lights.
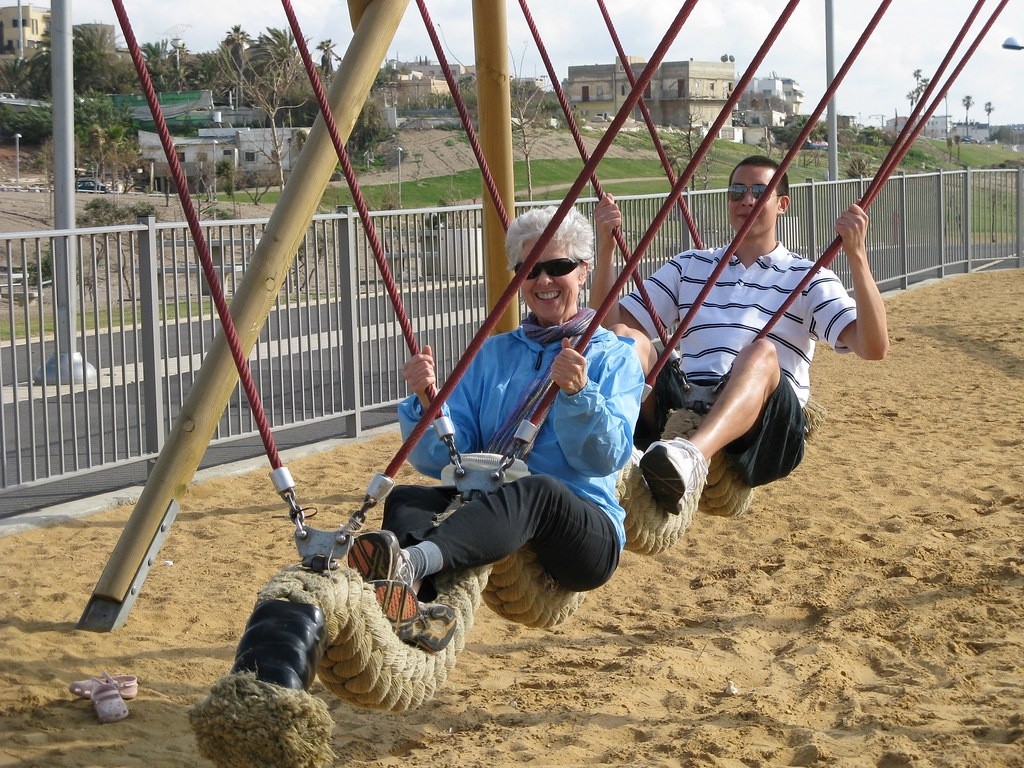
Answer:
[173,38,182,86]
[869,115,883,127]
[211,140,218,201]
[15,134,22,186]
[396,147,402,209]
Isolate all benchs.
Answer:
[134,264,246,297]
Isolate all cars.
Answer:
[75,180,111,194]
[787,134,828,149]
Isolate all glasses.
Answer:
[513,258,582,280]
[728,183,782,202]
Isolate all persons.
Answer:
[346,204,644,651]
[588,155,889,514]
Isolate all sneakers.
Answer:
[367,580,458,653]
[348,529,413,585]
[640,436,705,515]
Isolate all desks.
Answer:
[162,238,262,292]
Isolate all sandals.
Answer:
[90,685,129,724]
[69,673,138,700]
